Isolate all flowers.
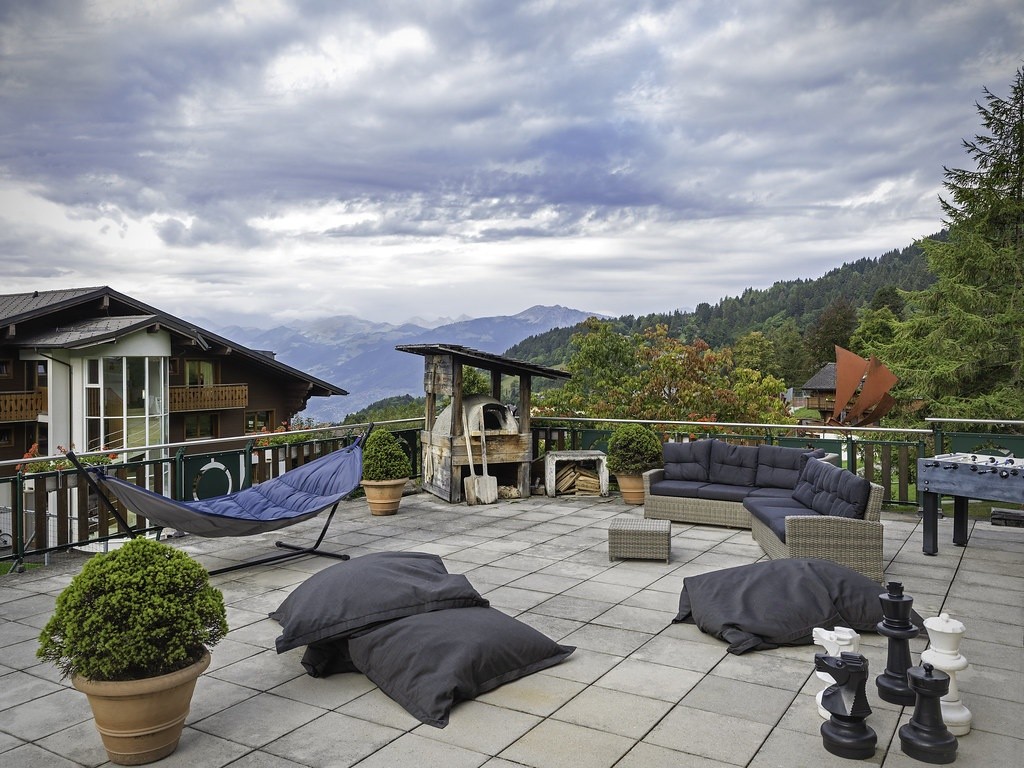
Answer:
[252,415,337,457]
[15,442,119,472]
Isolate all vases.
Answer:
[21,469,117,492]
[252,443,321,464]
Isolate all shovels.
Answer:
[461,405,498,505]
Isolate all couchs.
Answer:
[641,438,841,530]
[739,458,885,583]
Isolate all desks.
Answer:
[545,449,609,498]
[915,452,1024,557]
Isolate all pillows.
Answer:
[827,470,871,519]
[754,444,814,490]
[794,448,827,489]
[710,438,760,487]
[664,439,713,480]
[810,463,848,514]
[269,550,491,653]
[675,555,927,656]
[303,637,362,677]
[791,457,833,509]
[347,606,576,728]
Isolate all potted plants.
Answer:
[34,534,231,766]
[359,428,412,515]
[606,423,663,505]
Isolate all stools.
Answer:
[608,518,672,564]
